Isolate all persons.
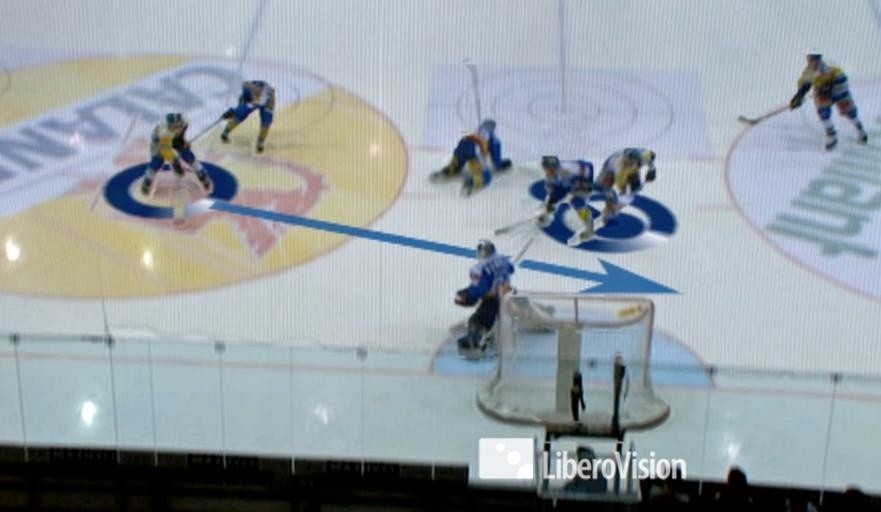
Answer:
[835,487,877,510]
[595,146,658,233]
[782,488,818,512]
[535,154,597,241]
[706,468,756,511]
[427,119,513,199]
[451,238,516,353]
[220,80,276,154]
[646,468,691,511]
[138,113,212,198]
[789,53,870,152]
[563,442,609,493]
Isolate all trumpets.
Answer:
[739,106,787,125]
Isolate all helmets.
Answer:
[622,147,640,165]
[476,238,495,258]
[542,156,559,170]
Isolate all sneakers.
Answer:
[257,137,265,153]
[221,127,230,140]
[197,169,211,190]
[577,227,595,238]
[826,136,838,149]
[856,124,867,140]
[142,176,152,194]
[459,334,490,351]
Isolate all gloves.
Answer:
[172,160,183,175]
[790,94,803,108]
[645,164,655,181]
[223,108,238,119]
